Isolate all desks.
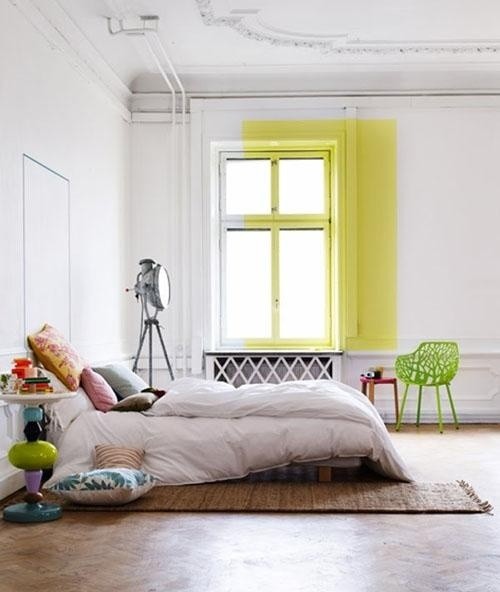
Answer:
[0,392,77,523]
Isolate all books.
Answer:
[21,376,52,393]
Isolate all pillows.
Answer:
[82,367,118,414]
[94,444,145,470]
[93,363,152,398]
[36,366,96,430]
[48,469,161,505]
[113,392,158,411]
[28,324,91,392]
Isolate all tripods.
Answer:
[131,319,176,390]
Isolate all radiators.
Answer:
[226,363,330,388]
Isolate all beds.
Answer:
[42,379,413,489]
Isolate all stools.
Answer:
[360,377,398,423]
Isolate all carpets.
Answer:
[0,480,495,513]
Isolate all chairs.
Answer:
[395,341,460,433]
